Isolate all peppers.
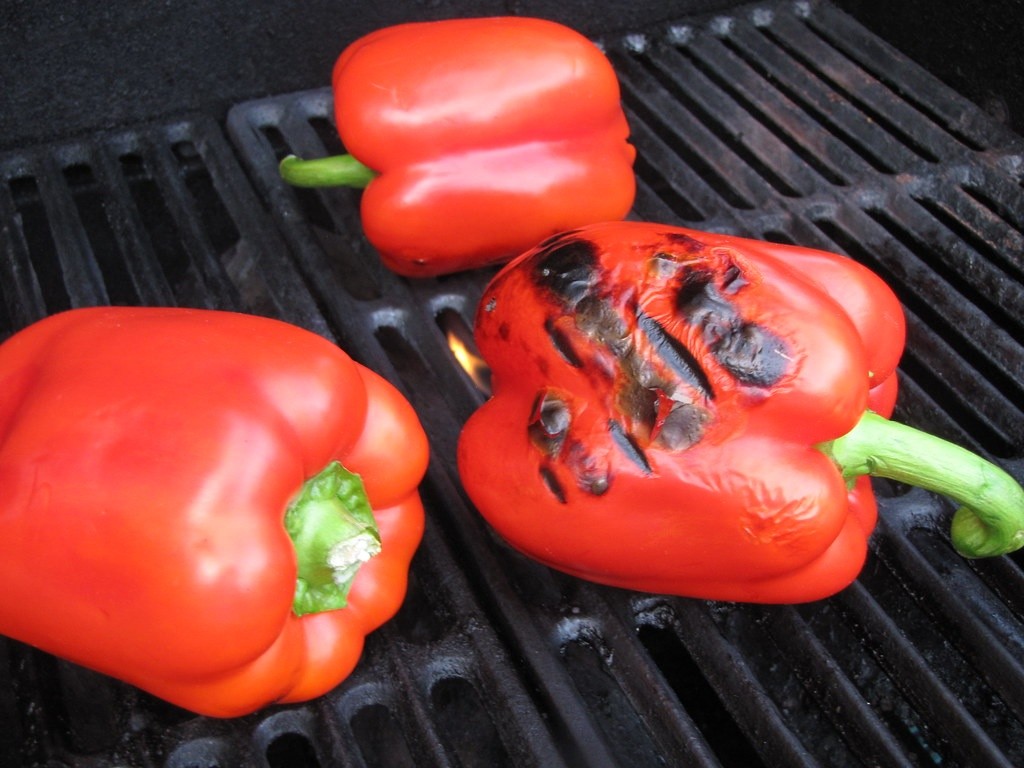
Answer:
[1,305,429,720]
[458,221,1022,653]
[281,20,636,276]
